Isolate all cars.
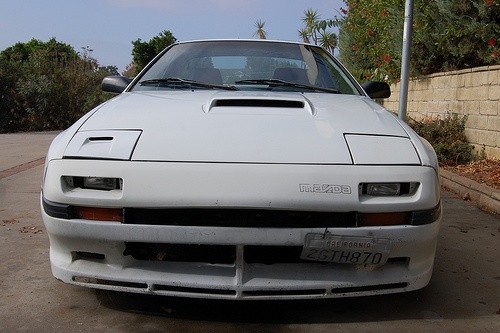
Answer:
[42,38,443,300]
